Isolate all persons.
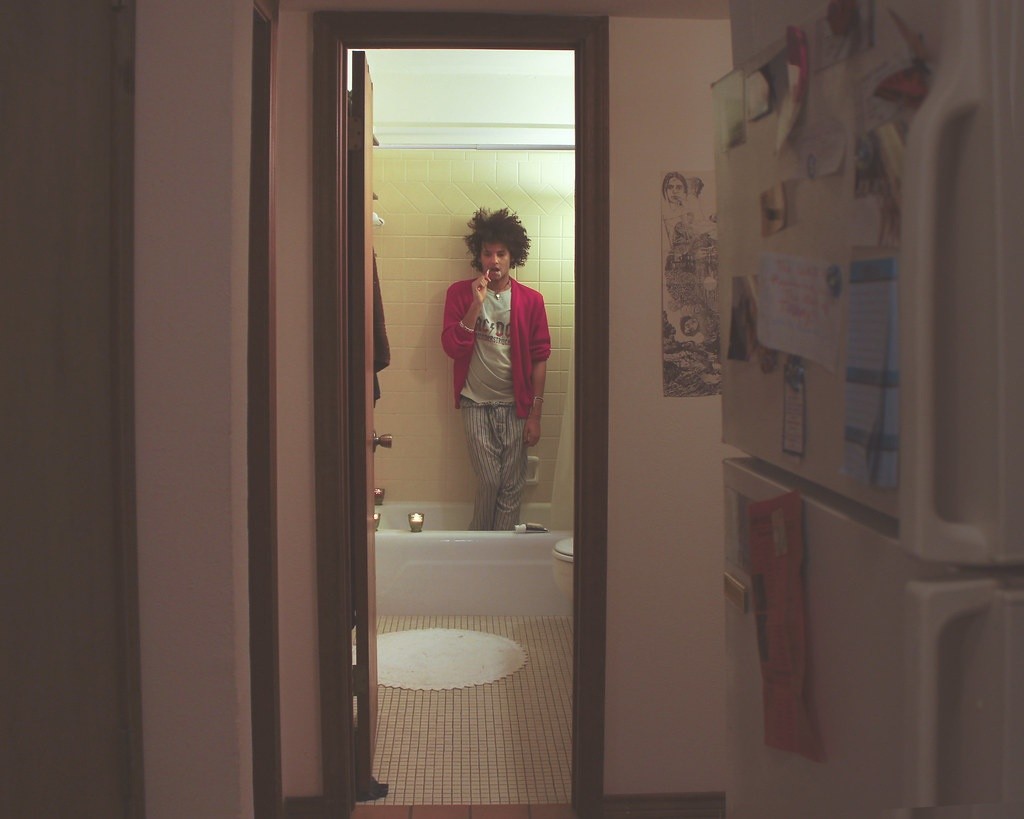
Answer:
[438,205,551,532]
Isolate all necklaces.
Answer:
[488,279,510,299]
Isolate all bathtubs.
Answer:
[374,501,575,617]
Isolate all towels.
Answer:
[373,249,390,408]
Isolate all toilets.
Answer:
[552,536,574,606]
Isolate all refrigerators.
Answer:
[714,0,1024,819]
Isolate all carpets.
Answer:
[351,627,528,692]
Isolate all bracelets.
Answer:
[458,319,475,333]
[533,396,543,403]
